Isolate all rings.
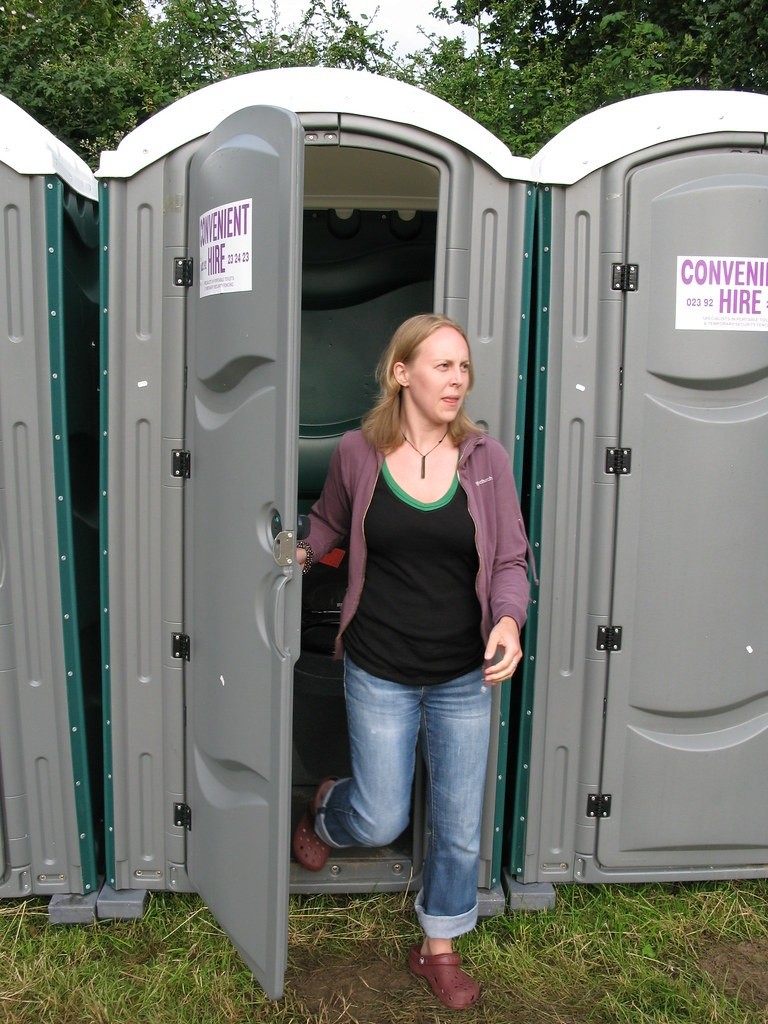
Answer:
[511,660,518,667]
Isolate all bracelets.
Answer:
[296,541,314,575]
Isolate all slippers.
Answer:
[291,773,342,870]
[407,943,479,1010]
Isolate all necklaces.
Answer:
[400,430,448,479]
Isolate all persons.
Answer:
[295,315,540,1009]
